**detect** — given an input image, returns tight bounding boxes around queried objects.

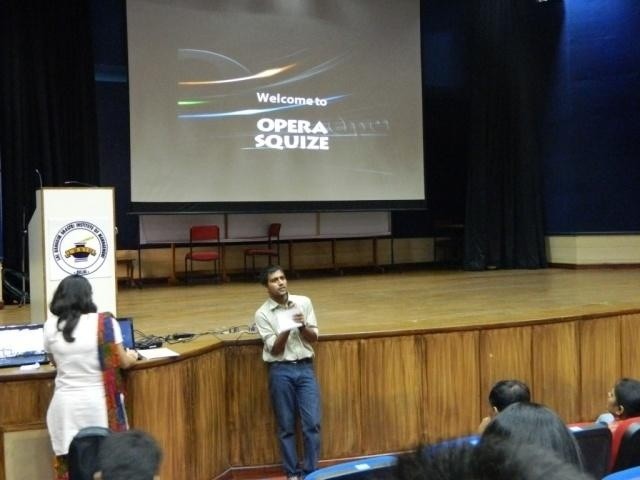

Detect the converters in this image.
[172,331,193,340]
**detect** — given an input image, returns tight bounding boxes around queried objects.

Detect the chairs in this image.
[306,456,397,480]
[184,225,221,280]
[612,414,639,472]
[243,222,281,277]
[565,421,610,478]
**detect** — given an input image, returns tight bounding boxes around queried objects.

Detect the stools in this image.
[117,258,135,286]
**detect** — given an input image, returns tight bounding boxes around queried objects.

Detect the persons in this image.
[480,402,585,471]
[366,433,598,480]
[93,429,163,480]
[254,264,321,480]
[44,275,138,480]
[607,378,640,437]
[478,380,531,432]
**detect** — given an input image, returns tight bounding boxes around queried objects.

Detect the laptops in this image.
[111,316,136,361]
[0,325,53,368]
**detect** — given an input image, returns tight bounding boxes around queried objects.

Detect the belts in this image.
[283,359,312,364]
